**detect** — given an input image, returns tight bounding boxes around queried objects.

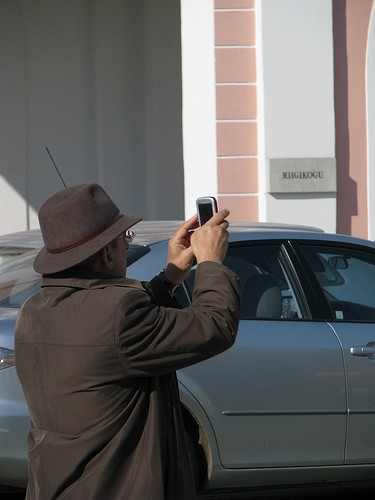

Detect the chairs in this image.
[185,269,196,303]
[242,274,283,318]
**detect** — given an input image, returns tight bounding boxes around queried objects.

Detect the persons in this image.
[13,184,242,499]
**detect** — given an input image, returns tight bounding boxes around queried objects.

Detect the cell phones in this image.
[196,197,217,227]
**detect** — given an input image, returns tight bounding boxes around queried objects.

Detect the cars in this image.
[0,219,375,500]
